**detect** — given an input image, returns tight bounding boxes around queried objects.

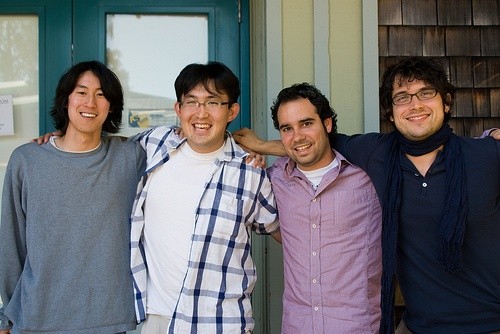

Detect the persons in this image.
[174,83,500,334]
[233,57,500,334]
[0,61,266,334]
[31,61,283,334]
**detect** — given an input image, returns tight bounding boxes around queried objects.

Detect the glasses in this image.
[392,88,438,106]
[179,99,230,112]
